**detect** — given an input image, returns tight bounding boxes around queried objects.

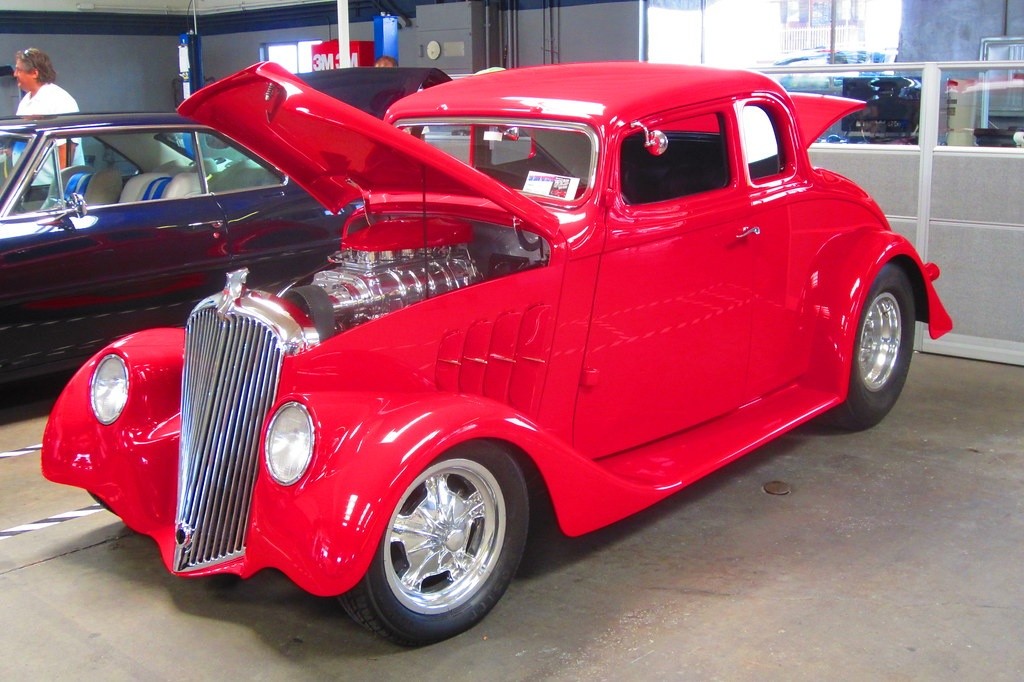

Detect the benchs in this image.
[621,129,726,204]
[158,157,267,192]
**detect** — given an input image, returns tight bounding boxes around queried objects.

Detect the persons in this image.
[0,46,86,202]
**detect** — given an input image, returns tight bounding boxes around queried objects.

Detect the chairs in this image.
[119,172,204,204]
[39,167,124,210]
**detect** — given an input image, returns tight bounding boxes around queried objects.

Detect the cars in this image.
[41,55,953,649]
[0,64,597,389]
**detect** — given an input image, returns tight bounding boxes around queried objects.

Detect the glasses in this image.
[24,49,36,68]
